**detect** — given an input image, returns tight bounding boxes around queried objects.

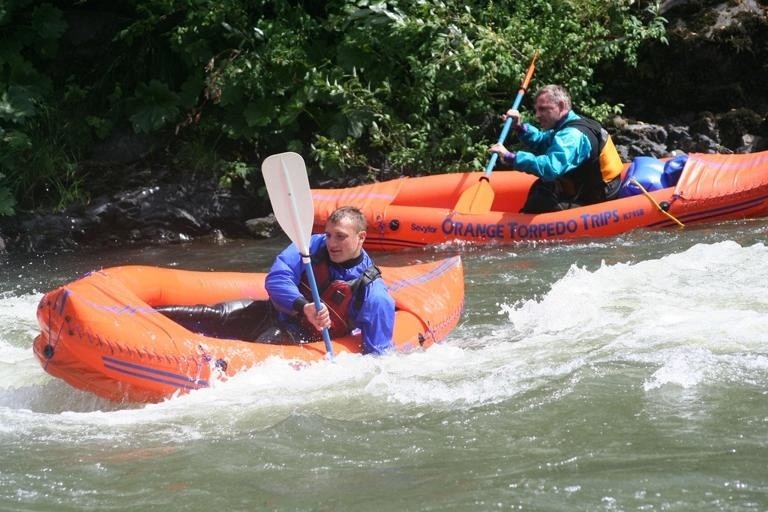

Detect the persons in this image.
[486,83,624,215]
[153,204,398,357]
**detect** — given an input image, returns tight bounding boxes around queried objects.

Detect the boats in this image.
[33,255,464,404]
[309,150,768,251]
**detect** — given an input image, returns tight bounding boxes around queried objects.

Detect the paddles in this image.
[261,152,334,361]
[454,53,538,212]
